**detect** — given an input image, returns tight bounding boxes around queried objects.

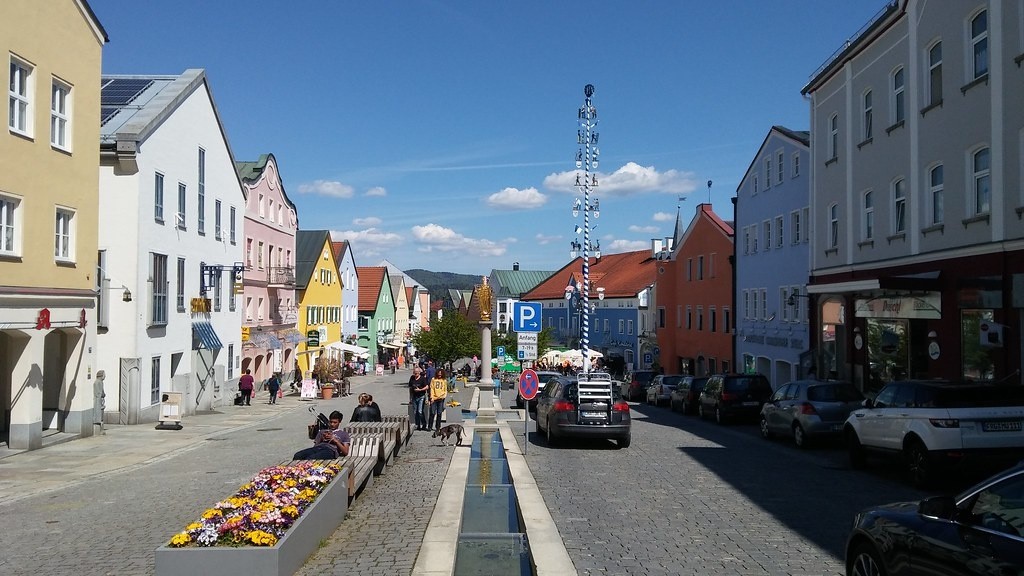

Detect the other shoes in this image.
[273,401,275,404]
[416,423,420,430]
[246,403,251,406]
[436,427,440,433]
[422,423,427,430]
[428,427,432,431]
[269,400,271,404]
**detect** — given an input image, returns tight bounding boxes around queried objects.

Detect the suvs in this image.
[697,372,775,425]
[625,369,664,403]
[843,376,1024,489]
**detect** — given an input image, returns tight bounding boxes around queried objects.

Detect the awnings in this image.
[388,342,407,348]
[332,343,370,355]
[260,332,282,347]
[354,352,371,359]
[278,330,301,344]
[193,323,225,351]
[380,344,398,350]
[290,328,310,343]
[248,335,271,350]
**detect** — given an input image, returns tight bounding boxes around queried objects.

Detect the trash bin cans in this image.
[158,390,182,425]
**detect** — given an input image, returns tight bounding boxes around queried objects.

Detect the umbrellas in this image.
[545,347,605,365]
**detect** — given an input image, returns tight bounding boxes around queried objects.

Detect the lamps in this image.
[109,285,131,301]
[787,293,808,306]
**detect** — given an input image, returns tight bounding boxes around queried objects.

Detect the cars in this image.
[647,374,687,407]
[516,370,563,409]
[669,376,710,416]
[534,376,630,448]
[845,460,1024,576]
[760,378,865,450]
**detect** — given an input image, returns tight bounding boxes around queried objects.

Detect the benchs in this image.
[280,414,415,505]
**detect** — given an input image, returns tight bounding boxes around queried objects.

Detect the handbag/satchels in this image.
[308,424,319,439]
[250,389,255,398]
[277,388,282,398]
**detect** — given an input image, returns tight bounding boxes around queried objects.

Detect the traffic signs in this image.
[497,346,505,356]
[513,302,542,333]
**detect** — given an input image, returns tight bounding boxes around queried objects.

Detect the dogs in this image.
[432,424,467,446]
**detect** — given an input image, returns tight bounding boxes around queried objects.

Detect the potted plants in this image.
[321,383,334,399]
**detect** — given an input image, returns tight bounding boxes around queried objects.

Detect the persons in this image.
[239,370,255,406]
[348,393,382,424]
[414,355,436,405]
[312,370,321,389]
[461,354,502,379]
[266,374,280,403]
[408,367,448,429]
[291,365,302,391]
[382,351,419,372]
[536,361,583,376]
[293,411,351,460]
